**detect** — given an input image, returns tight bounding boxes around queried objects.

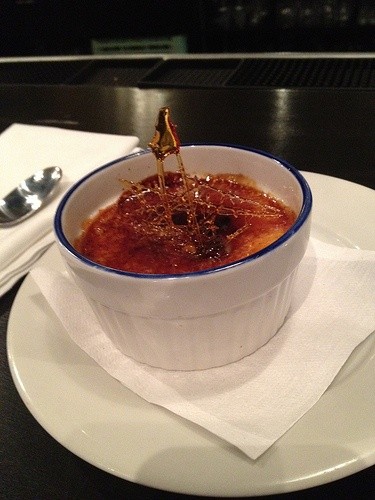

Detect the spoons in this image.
[0,166,61,228]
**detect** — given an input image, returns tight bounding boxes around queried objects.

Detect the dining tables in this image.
[0,76,375,500]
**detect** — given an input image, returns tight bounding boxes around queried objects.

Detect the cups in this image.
[56,139,314,375]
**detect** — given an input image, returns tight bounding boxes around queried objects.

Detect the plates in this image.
[6,166,374,500]
